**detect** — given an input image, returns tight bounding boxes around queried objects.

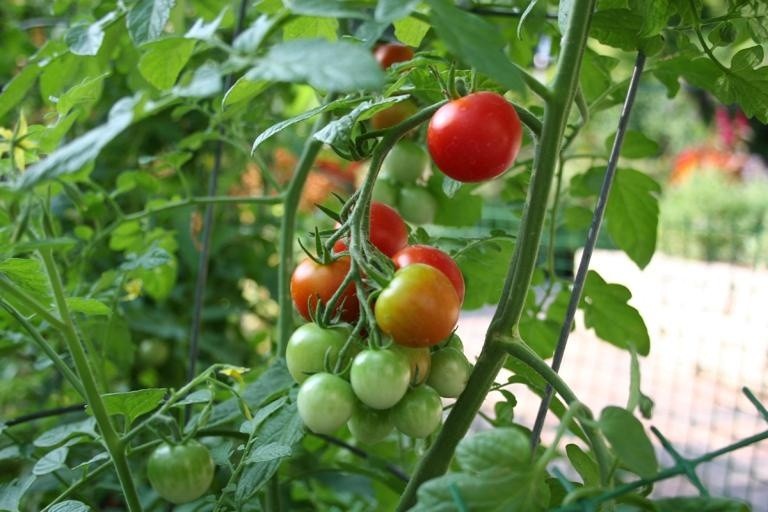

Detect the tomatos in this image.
[138,340,167,366]
[148,438,213,505]
[284,43,522,445]
